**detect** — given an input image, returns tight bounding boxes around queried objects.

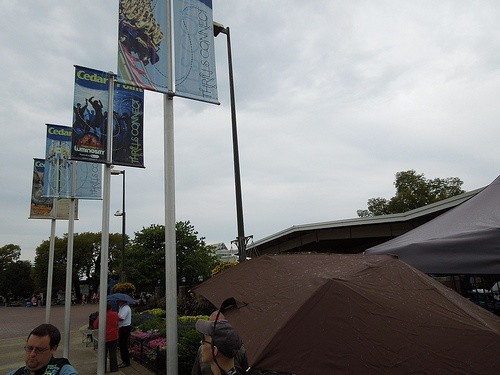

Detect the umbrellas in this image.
[188,254,500,375]
[107,294,135,305]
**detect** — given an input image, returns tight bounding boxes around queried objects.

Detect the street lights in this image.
[111,169,126,284]
[212,21,249,263]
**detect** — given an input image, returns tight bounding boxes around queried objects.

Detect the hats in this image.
[195,319,242,356]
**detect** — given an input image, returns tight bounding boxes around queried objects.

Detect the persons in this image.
[7,323,78,375]
[191,310,248,375]
[93,305,119,373]
[116,300,132,367]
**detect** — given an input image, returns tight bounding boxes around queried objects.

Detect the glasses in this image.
[24,343,51,353]
[202,335,216,346]
